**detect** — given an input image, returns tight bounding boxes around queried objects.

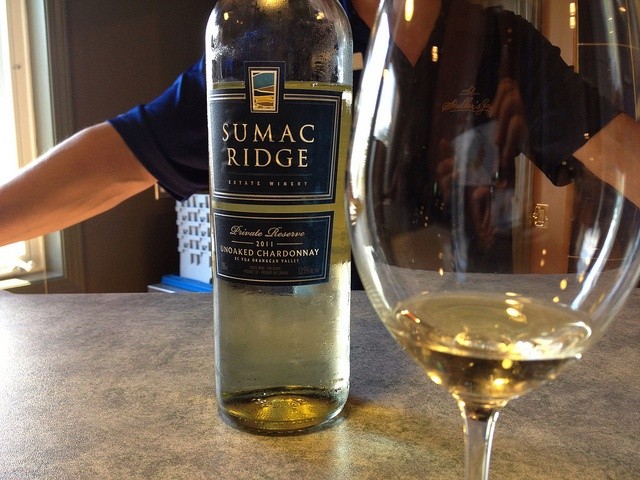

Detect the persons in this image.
[0,0,640,251]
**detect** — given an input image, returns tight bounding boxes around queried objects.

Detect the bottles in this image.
[205,0,352,440]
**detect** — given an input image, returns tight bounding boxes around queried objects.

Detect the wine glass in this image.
[344,0,640,480]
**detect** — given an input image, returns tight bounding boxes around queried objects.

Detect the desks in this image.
[1,290,640,480]
